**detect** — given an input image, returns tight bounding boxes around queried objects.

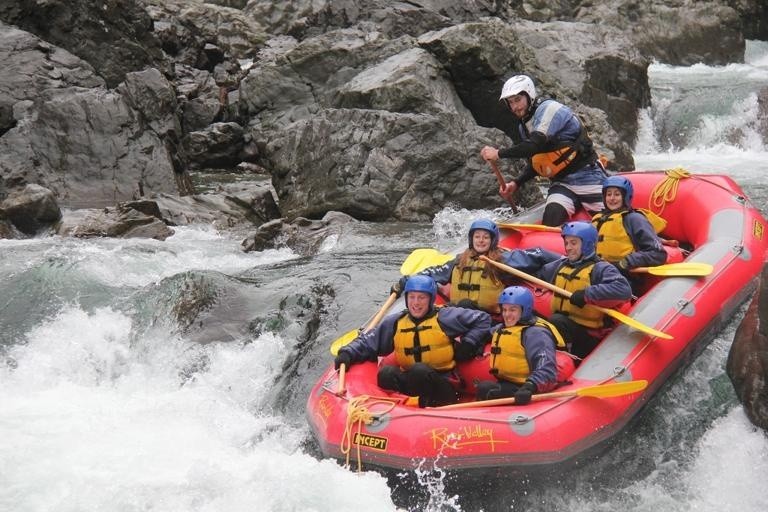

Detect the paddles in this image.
[363,248,440,333]
[329,256,452,357]
[627,263,714,277]
[498,223,678,248]
[480,256,673,341]
[425,380,647,409]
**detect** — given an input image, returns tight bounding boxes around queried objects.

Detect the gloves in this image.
[335,352,353,371]
[570,289,586,308]
[455,343,473,362]
[390,283,402,299]
[617,259,631,278]
[515,383,534,404]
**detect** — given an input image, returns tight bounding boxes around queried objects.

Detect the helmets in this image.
[561,221,598,257]
[468,219,499,251]
[499,75,537,104]
[602,176,634,208]
[405,275,437,312]
[498,285,534,320]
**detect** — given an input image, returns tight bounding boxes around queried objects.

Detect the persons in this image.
[586,172,667,281]
[477,72,614,229]
[334,272,493,406]
[475,283,560,408]
[384,216,525,313]
[494,220,631,346]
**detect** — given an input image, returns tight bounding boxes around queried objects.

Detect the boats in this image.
[305,171,768,491]
